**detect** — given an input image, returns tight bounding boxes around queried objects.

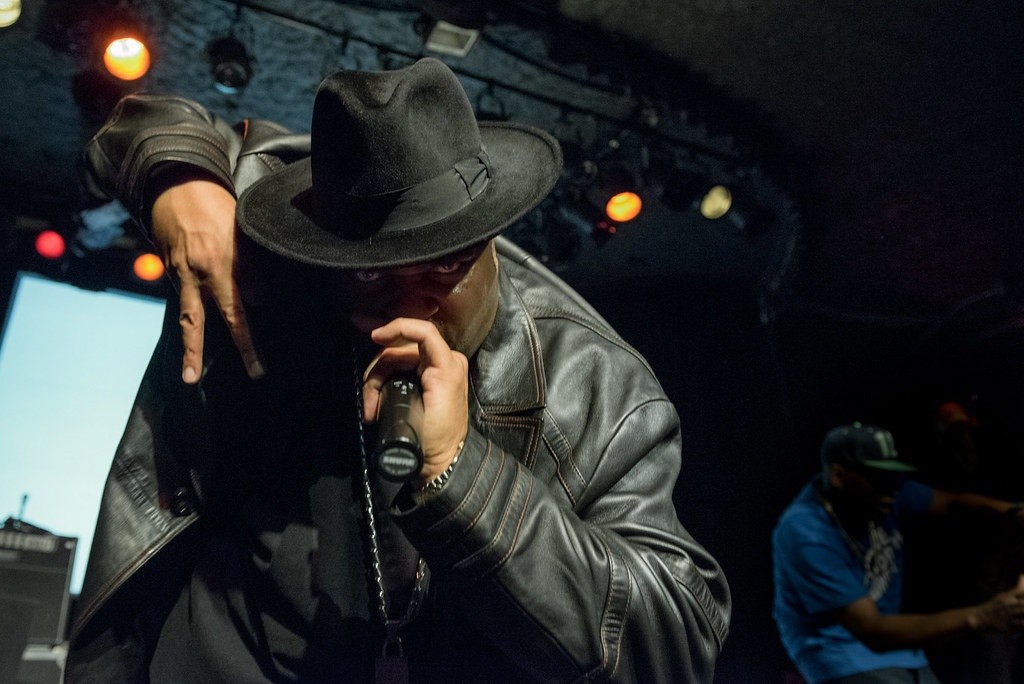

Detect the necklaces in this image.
[350,338,429,683]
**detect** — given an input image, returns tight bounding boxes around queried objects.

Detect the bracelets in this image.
[414,439,467,506]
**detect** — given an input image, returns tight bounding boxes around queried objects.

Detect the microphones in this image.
[372,370,427,484]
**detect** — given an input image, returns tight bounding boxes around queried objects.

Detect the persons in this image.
[768,423,1024,684]
[78,55,730,684]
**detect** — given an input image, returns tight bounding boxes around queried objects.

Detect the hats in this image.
[821,422,918,472]
[234,56,563,267]
